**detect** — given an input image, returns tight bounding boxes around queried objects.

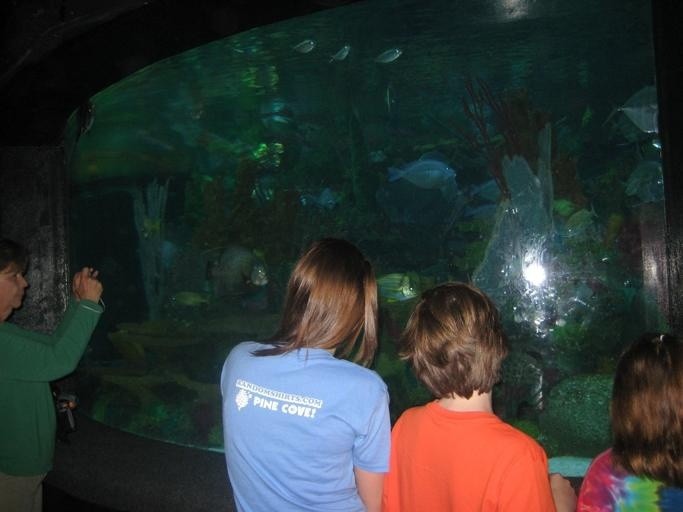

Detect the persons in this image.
[219,237,392,512]
[381,283,578,512]
[575,332,683,512]
[0,239,105,512]
